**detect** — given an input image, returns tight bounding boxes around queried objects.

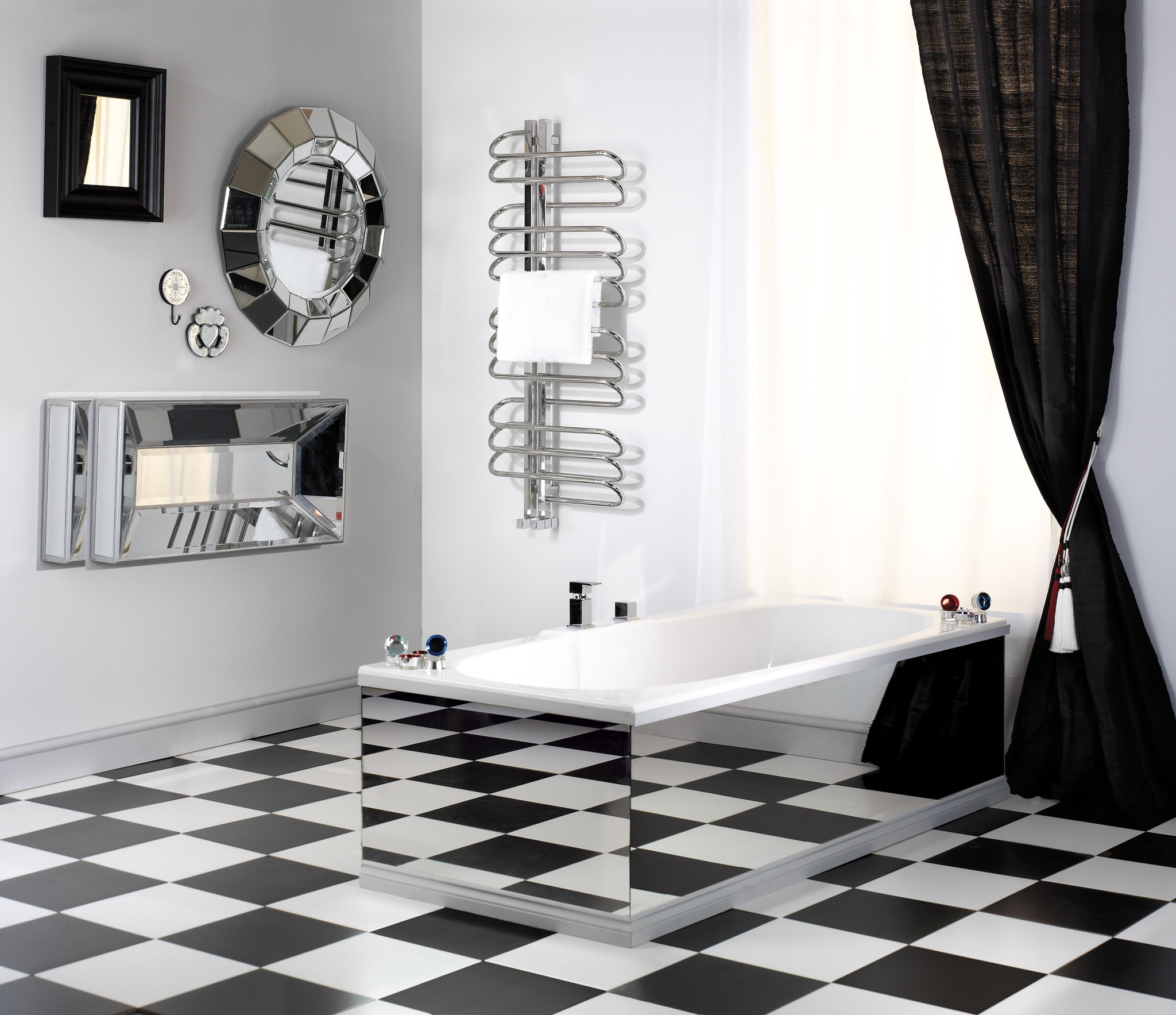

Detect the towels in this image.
[496,269,600,364]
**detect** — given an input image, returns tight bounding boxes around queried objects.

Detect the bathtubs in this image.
[359,595,1005,947]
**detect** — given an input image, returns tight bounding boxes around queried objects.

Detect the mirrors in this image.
[44,55,166,224]
[219,107,389,347]
